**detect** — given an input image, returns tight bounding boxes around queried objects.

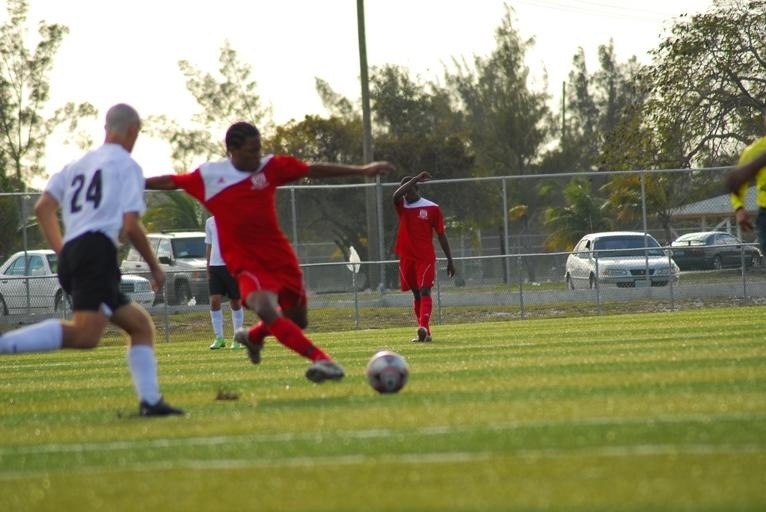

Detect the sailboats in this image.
[366,351,411,391]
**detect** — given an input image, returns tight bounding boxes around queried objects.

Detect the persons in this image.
[726,135,766,258]
[200,215,243,350]
[389,171,456,343]
[131,119,396,386]
[0,101,183,417]
[725,153,766,196]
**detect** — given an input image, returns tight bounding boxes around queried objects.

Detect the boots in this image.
[116,228,228,308]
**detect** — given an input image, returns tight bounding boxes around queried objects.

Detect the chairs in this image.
[210,338,224,349]
[411,328,433,342]
[231,326,263,363]
[139,397,183,416]
[307,359,343,383]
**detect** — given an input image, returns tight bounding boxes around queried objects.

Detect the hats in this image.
[661,229,762,273]
[0,245,159,318]
[562,230,681,290]
